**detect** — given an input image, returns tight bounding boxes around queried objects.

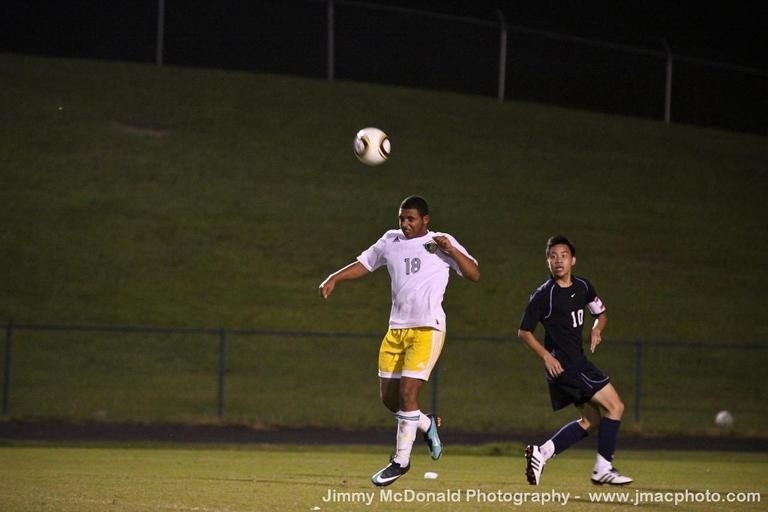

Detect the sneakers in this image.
[371,458,411,488]
[591,467,633,485]
[423,413,444,460]
[525,444,546,486]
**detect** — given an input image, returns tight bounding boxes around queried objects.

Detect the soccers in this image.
[352,127,391,168]
[715,410,734,428]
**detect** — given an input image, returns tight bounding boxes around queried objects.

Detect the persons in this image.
[318,195,482,488]
[515,232,637,486]
